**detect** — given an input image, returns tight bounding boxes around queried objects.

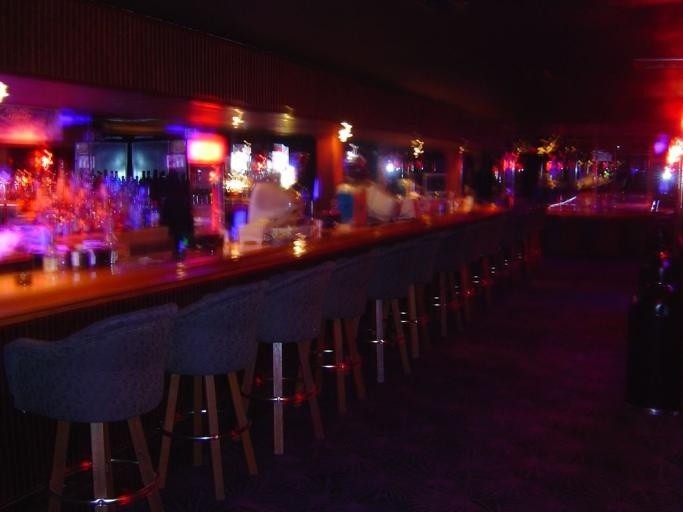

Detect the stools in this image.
[153,281,268,501]
[351,216,525,383]
[2,301,181,510]
[243,264,335,456]
[293,253,379,411]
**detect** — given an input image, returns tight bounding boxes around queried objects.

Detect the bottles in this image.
[90,165,169,206]
